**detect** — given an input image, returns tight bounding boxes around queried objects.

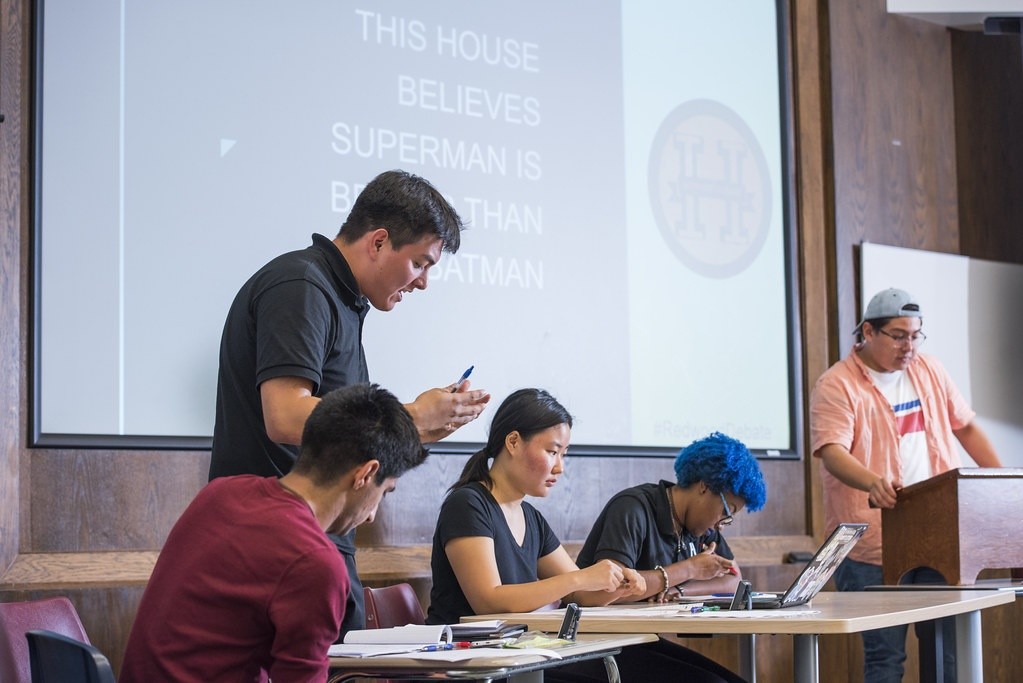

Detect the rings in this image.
[446,422,452,431]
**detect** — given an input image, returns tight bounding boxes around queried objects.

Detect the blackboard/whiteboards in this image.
[859,240,1023,468]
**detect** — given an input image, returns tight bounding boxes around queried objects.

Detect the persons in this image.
[208,171,490,630]
[120,383,425,683]
[808,289,1001,683]
[574,432,765,683]
[425,389,648,683]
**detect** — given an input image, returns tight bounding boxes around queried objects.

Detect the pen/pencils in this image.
[421,644,454,651]
[701,544,739,576]
[450,366,474,391]
[691,606,721,614]
[453,642,471,648]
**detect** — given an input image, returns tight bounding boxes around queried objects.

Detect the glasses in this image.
[714,484,734,527]
[872,324,927,349]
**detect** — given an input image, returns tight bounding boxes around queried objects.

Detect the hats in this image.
[852,287,923,336]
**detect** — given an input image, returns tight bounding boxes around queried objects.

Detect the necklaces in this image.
[666,488,681,554]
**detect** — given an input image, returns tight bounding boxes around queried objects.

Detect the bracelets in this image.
[655,565,669,595]
[675,585,685,596]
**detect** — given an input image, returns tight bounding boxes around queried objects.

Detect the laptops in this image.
[702,524,869,606]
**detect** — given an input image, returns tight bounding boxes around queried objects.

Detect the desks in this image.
[459,589,1016,683]
[322,632,658,683]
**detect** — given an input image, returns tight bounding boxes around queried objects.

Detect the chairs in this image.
[0,595,116,683]
[362,582,428,632]
